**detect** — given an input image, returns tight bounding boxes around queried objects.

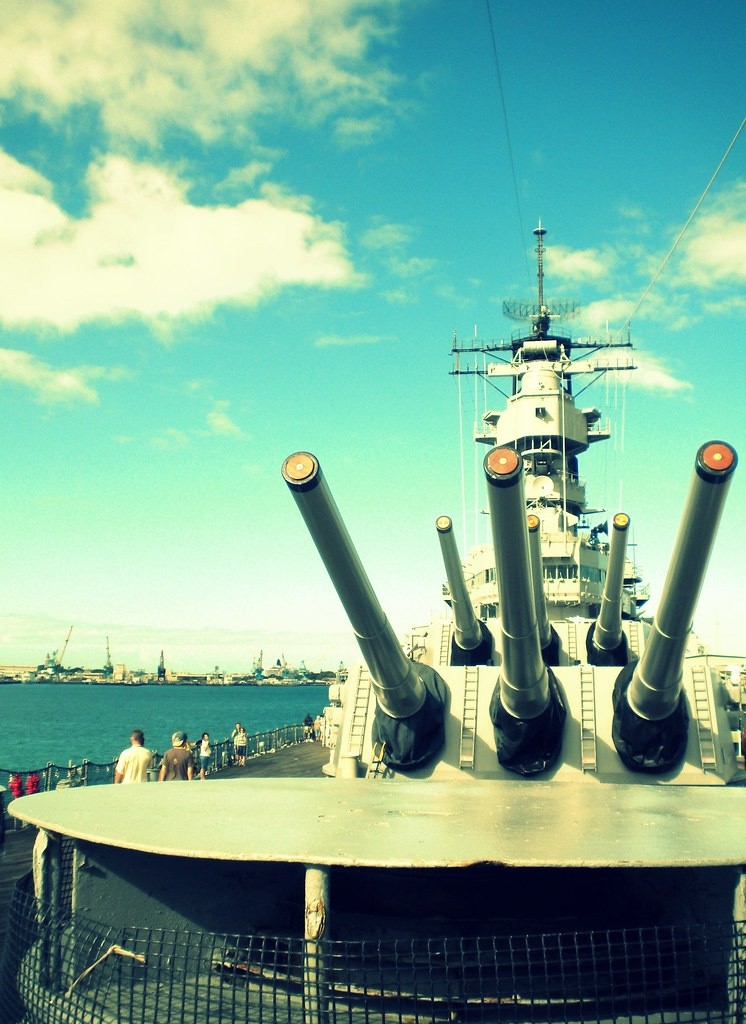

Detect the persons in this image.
[231,722,248,767]
[113,729,153,783]
[302,713,325,743]
[158,731,195,782]
[193,733,212,780]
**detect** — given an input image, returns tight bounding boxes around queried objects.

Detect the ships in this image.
[10,219,746,1024]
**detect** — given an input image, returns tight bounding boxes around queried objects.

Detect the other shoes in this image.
[233,762,246,768]
[305,738,313,743]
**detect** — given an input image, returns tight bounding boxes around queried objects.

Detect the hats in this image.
[171,731,187,748]
